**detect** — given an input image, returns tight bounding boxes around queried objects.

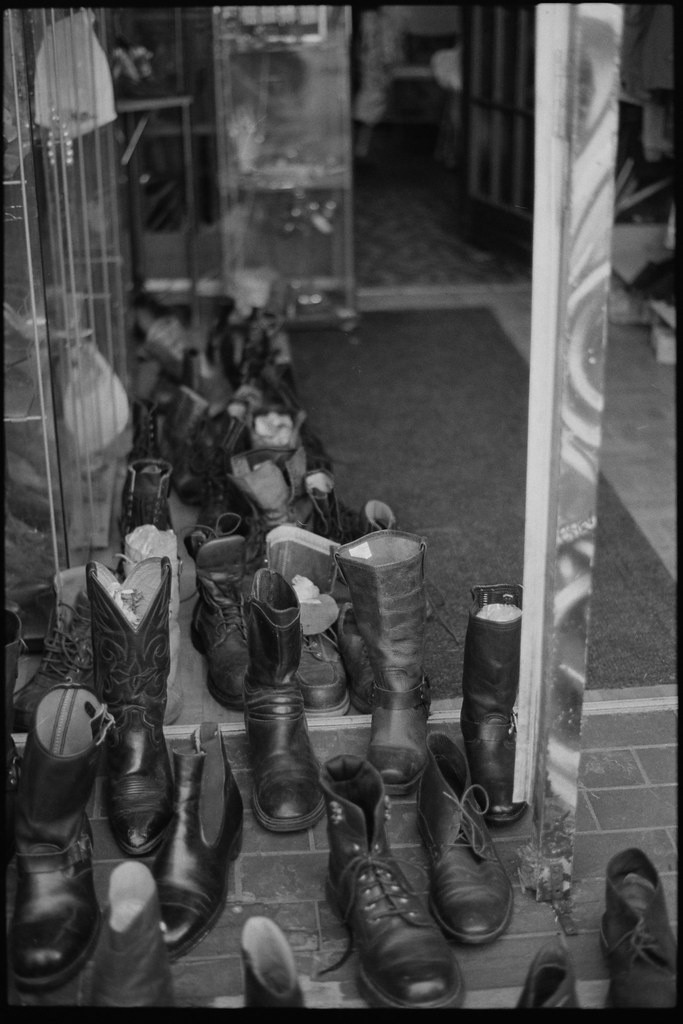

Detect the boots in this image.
[1,293,678,1009]
